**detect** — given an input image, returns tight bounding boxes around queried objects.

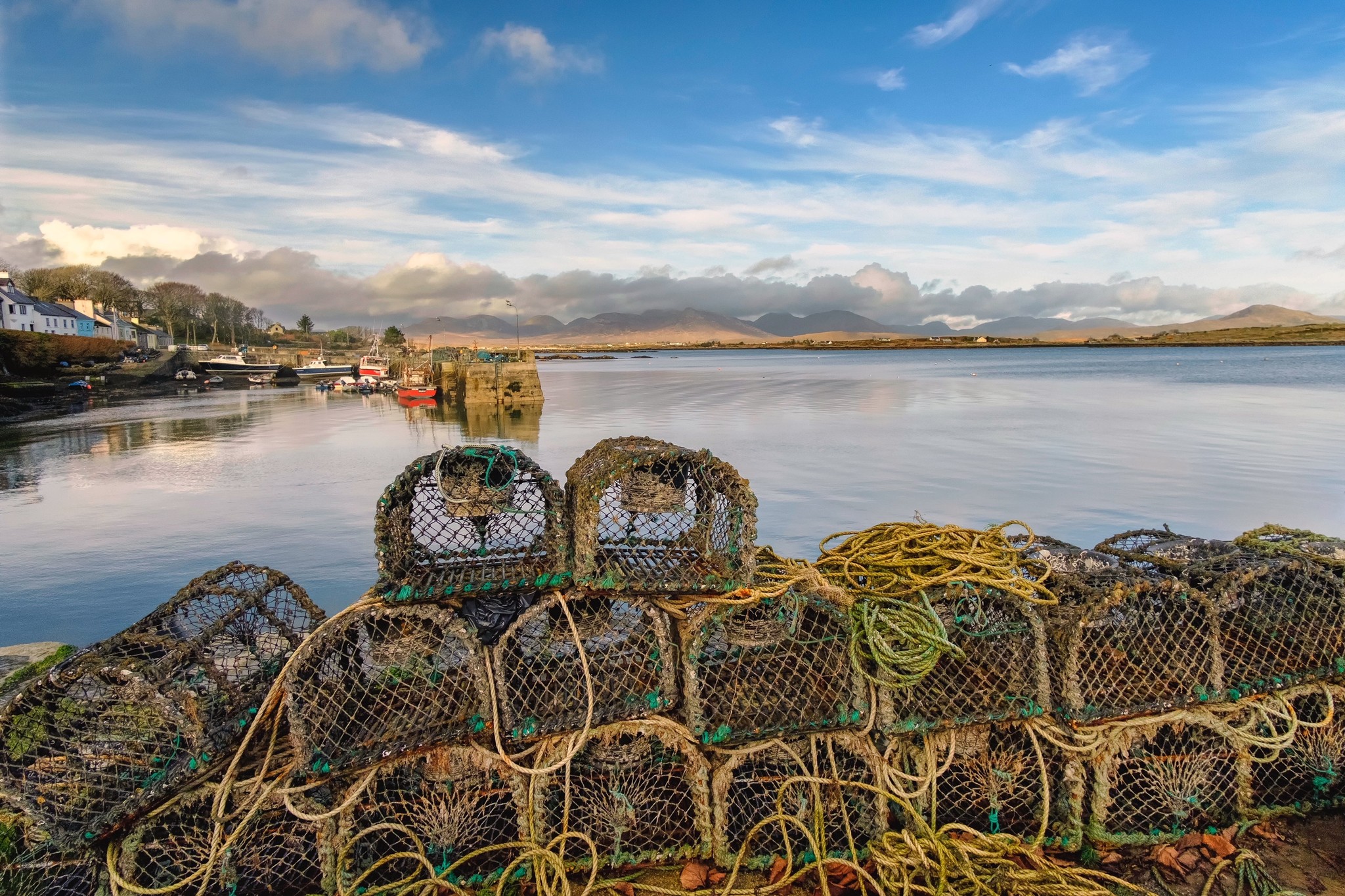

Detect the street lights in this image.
[436,317,447,351]
[506,299,520,350]
[401,324,410,350]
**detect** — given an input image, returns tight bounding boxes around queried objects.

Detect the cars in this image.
[177,344,189,350]
[137,355,147,363]
[198,344,211,351]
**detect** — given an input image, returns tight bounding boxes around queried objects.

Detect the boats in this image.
[332,376,358,392]
[208,376,223,385]
[395,334,437,398]
[290,339,354,380]
[357,319,390,379]
[356,376,379,394]
[174,367,197,380]
[315,378,334,390]
[68,380,92,390]
[378,380,398,392]
[196,354,286,378]
[247,372,275,383]
